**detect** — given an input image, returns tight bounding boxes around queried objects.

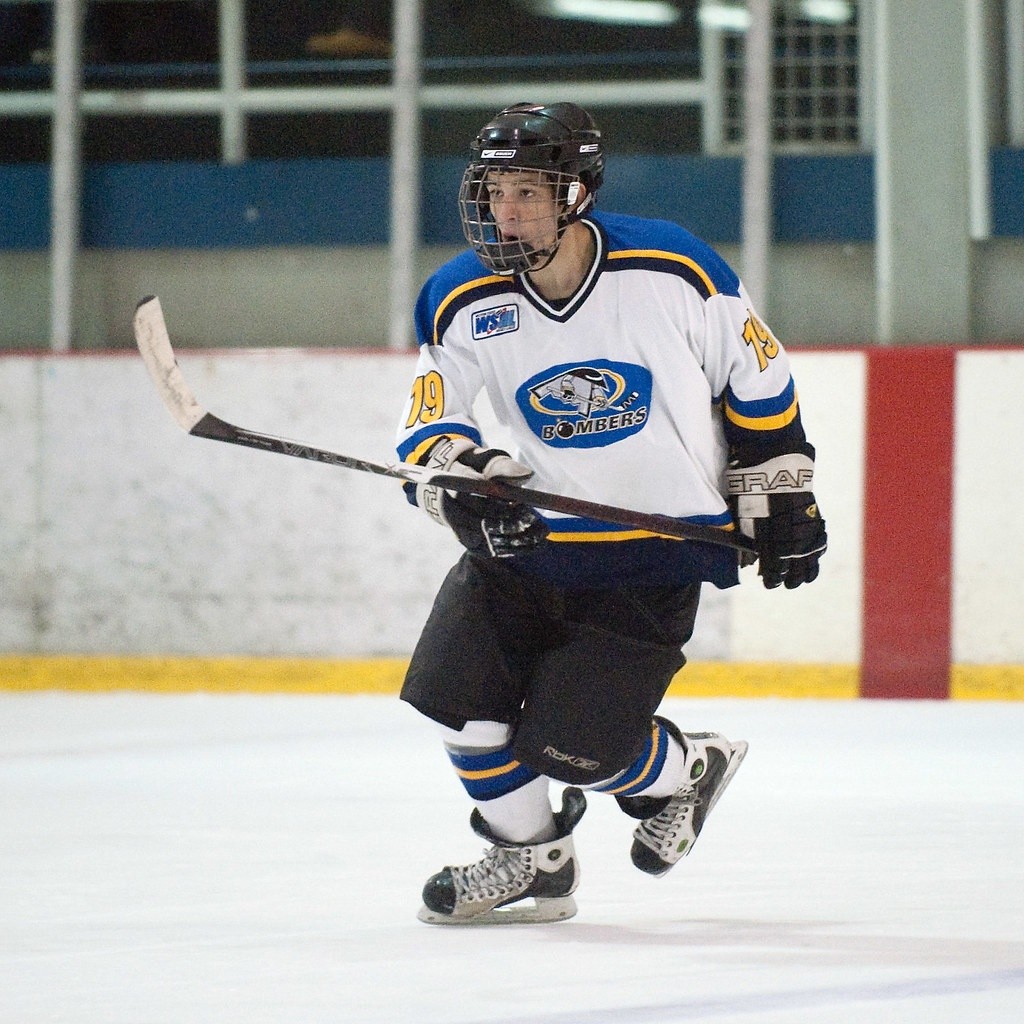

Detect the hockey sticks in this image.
[133,291,757,555]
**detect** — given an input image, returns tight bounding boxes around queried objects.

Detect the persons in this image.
[397,104,828,925]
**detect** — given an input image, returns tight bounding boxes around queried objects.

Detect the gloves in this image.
[726,488,827,589]
[415,443,551,562]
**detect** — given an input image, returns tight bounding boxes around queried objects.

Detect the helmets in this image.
[458,101,605,276]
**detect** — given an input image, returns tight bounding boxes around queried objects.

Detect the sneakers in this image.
[631,715,748,879]
[418,786,587,925]
